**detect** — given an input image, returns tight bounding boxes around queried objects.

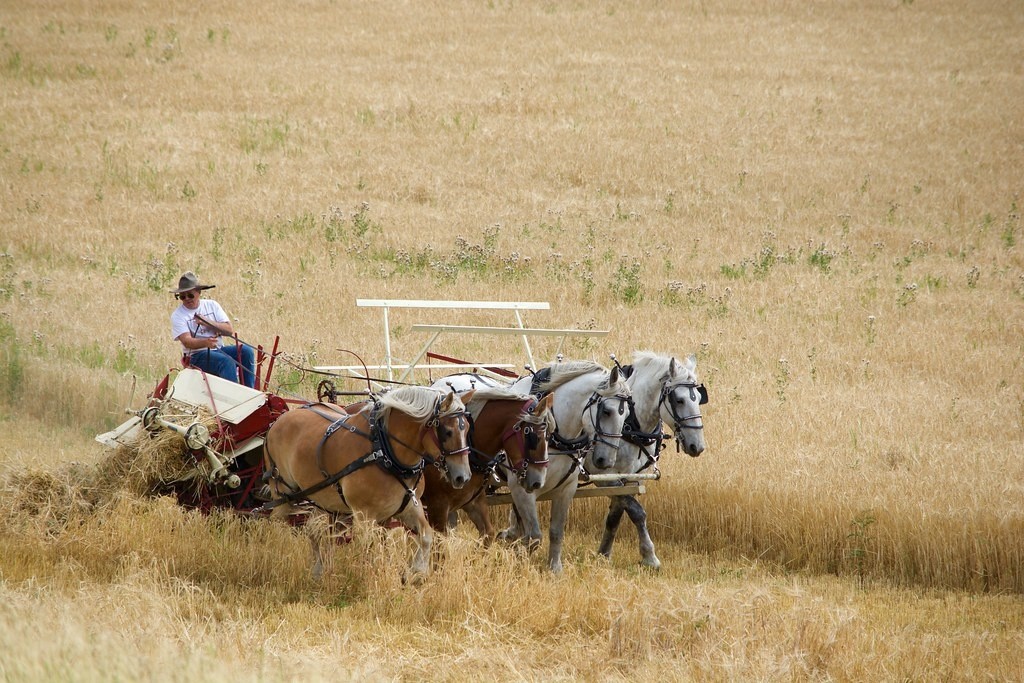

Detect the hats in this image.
[169,271,215,292]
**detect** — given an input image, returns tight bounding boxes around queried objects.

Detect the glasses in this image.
[179,292,198,300]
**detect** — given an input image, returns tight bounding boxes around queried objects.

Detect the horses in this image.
[263,351,705,588]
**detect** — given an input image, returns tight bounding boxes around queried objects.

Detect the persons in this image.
[170,271,255,389]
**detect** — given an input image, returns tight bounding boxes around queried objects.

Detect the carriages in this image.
[92,297,709,584]
